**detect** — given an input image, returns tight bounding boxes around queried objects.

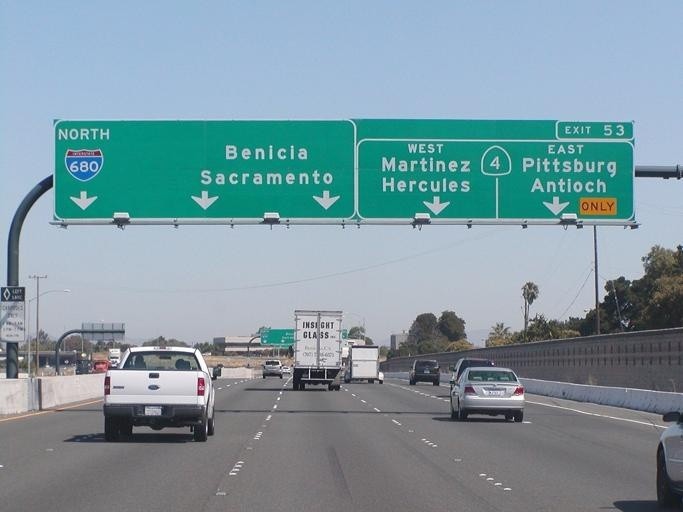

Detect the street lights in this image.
[26,285,70,301]
[27,273,49,375]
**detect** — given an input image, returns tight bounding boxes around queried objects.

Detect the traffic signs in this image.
[260,328,296,345]
[342,328,348,339]
[0,286,29,342]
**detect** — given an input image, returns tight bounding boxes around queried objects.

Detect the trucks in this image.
[341,345,381,384]
[1,343,122,377]
[294,309,344,391]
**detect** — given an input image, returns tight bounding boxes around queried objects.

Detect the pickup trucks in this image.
[99,346,222,442]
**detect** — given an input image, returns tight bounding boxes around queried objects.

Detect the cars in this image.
[282,364,291,375]
[654,407,683,512]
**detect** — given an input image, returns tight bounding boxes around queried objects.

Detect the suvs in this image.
[405,352,527,423]
[260,359,284,378]
